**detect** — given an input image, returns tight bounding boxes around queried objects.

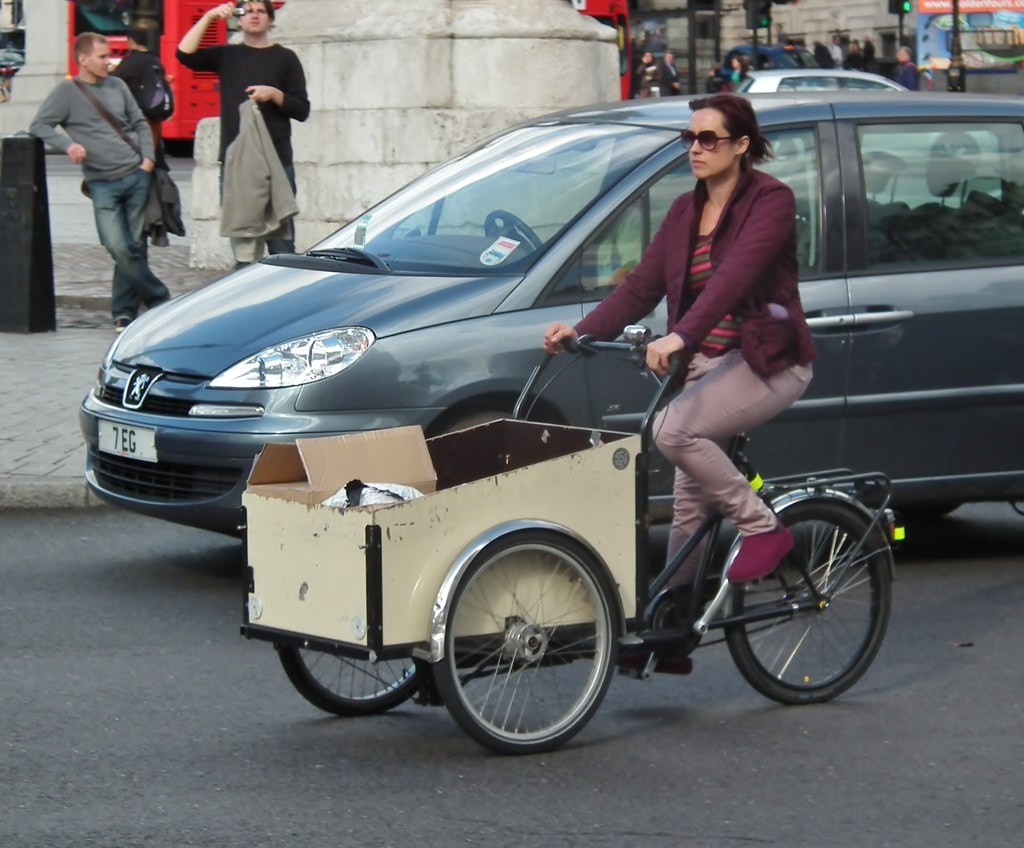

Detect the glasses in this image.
[680,128,733,151]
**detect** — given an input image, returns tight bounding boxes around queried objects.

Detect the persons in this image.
[179,0,310,271]
[627,26,921,96]
[32,29,175,332]
[537,95,819,676]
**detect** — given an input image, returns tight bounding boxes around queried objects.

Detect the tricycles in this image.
[235,322,907,758]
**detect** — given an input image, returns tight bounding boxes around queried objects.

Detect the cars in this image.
[719,39,822,83]
[70,86,1024,563]
[731,67,1001,181]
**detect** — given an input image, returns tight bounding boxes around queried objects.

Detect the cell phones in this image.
[233,8,245,17]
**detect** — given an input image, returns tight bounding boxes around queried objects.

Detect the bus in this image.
[61,0,637,158]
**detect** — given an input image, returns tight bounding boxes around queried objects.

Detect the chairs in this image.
[861,131,1024,264]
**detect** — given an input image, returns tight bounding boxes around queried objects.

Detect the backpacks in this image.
[130,51,174,121]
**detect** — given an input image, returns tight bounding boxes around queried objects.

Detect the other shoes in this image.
[113,317,133,332]
[727,526,795,583]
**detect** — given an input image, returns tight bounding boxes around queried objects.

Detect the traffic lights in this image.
[888,0,910,14]
[744,0,773,29]
